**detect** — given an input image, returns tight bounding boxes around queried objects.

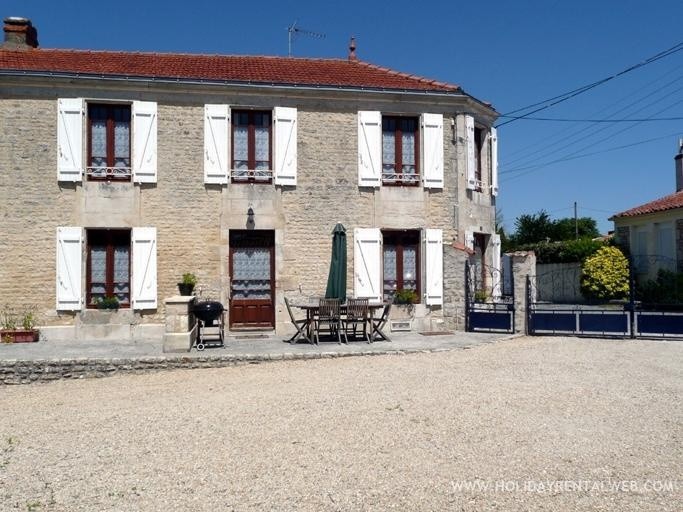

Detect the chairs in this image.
[283,295,395,344]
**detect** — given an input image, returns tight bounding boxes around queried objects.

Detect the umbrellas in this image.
[324,222,347,304]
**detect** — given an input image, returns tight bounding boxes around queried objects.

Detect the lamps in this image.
[245,207,255,224]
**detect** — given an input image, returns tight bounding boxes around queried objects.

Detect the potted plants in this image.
[0,307,40,345]
[175,273,197,296]
